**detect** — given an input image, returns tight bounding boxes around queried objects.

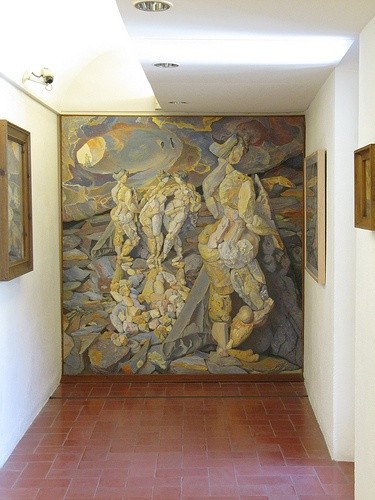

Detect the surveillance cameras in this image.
[41,67,53,84]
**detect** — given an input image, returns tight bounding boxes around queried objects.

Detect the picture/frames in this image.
[0,119,34,281]
[354,144,375,231]
[303,150,326,285]
